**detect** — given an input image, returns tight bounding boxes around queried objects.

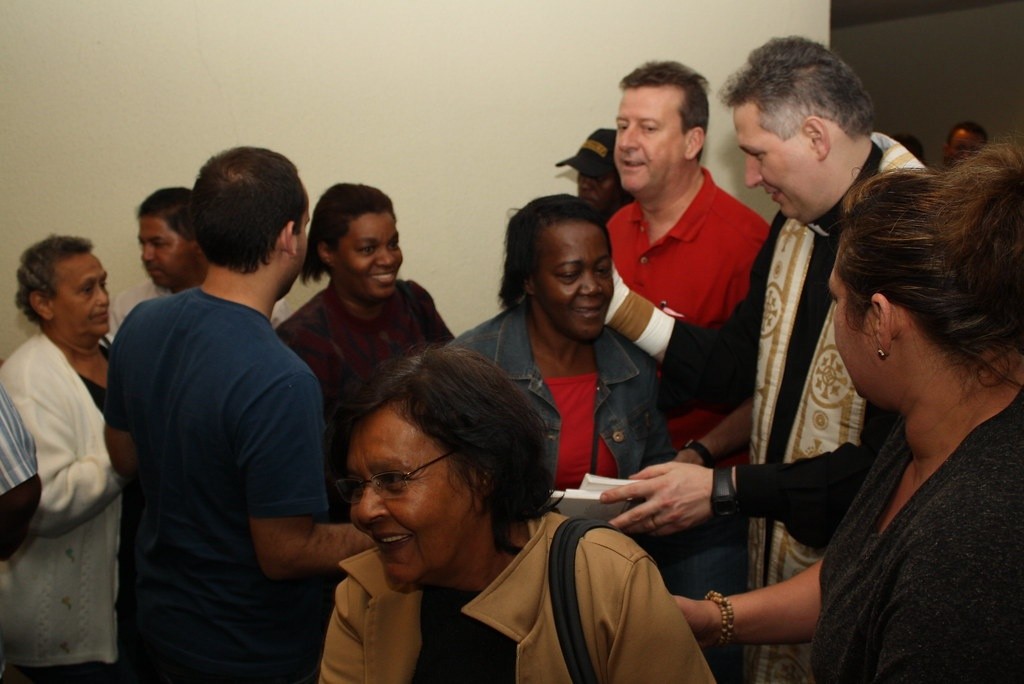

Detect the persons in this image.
[892,131,924,163]
[0,234,126,684]
[274,184,456,500]
[136,187,198,296]
[557,130,633,218]
[605,60,770,684]
[600,36,927,683]
[102,146,377,684]
[945,124,987,167]
[452,193,675,501]
[319,348,715,684]
[675,147,1024,684]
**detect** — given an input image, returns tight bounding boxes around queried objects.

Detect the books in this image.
[548,473,645,522]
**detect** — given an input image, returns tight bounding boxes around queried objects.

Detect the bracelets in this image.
[705,591,734,649]
[682,439,713,469]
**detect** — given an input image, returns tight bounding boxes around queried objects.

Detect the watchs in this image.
[710,466,739,520]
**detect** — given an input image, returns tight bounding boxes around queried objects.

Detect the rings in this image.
[651,515,660,529]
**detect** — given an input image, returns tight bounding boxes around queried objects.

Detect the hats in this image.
[556,129,616,177]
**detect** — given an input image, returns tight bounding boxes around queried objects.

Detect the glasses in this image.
[335,452,452,501]
